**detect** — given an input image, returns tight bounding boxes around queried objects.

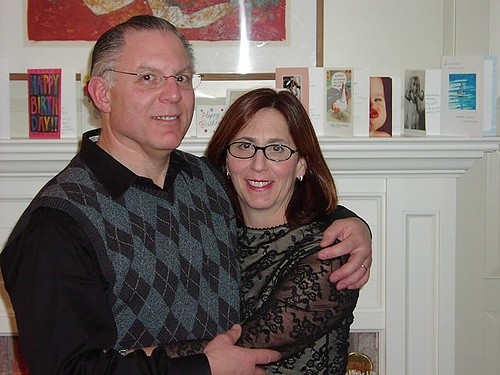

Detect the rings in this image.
[361,264,369,271]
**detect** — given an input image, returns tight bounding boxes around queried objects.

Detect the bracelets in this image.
[119,347,126,357]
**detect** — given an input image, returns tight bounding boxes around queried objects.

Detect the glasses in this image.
[101,68,204,90]
[226,141,298,162]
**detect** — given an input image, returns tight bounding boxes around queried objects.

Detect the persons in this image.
[116,88,360,375]
[369,78,391,137]
[0,15,372,375]
[406,77,425,131]
